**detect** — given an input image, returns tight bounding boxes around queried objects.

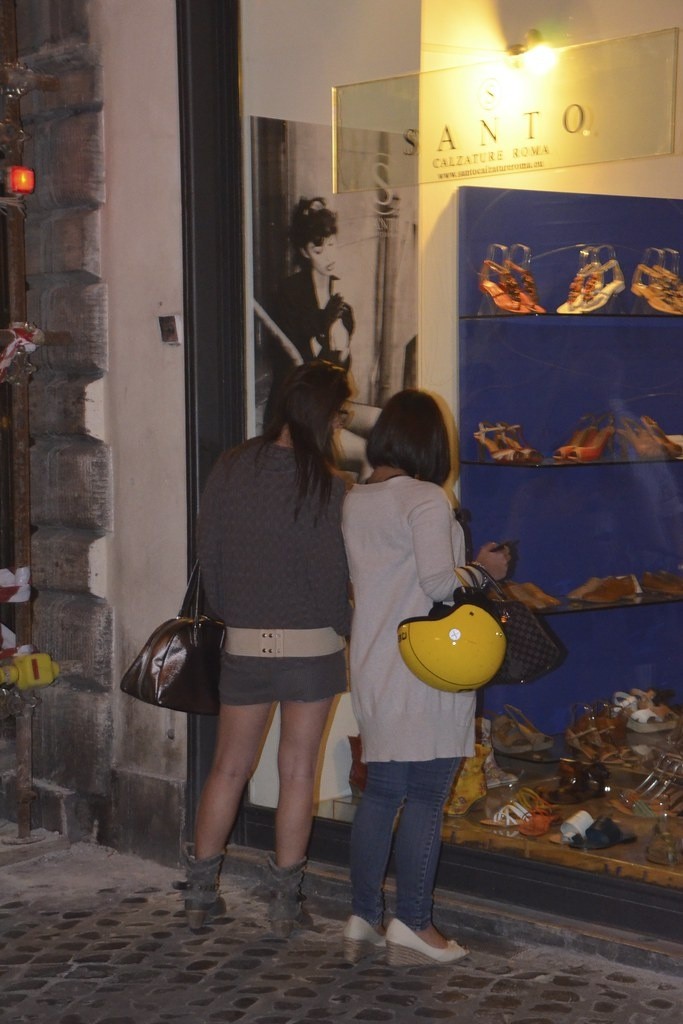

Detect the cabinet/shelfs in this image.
[454,185,683,782]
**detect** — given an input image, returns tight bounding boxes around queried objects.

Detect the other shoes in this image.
[476,688,683,866]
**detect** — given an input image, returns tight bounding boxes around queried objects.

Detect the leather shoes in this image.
[487,570,683,611]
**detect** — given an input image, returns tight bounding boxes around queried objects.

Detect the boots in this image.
[346,737,367,800]
[472,716,517,791]
[184,840,226,929]
[444,743,492,817]
[264,854,308,938]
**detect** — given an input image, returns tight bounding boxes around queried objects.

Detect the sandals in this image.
[579,259,625,313]
[479,260,531,314]
[499,260,546,315]
[556,262,604,315]
[631,264,683,316]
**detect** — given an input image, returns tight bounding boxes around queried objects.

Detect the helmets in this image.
[397,587,507,692]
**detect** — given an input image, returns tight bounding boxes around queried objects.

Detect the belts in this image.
[224,623,347,659]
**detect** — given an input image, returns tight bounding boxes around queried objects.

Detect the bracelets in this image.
[473,561,487,589]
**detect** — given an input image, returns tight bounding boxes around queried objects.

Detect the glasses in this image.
[334,408,349,426]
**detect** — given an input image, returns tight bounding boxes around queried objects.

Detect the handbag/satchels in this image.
[119,559,226,716]
[459,563,566,696]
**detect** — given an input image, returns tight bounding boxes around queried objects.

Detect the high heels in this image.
[343,915,384,964]
[471,414,682,466]
[385,917,471,968]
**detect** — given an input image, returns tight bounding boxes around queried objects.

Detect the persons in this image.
[341,388,511,968]
[262,197,385,482]
[185,358,352,943]
